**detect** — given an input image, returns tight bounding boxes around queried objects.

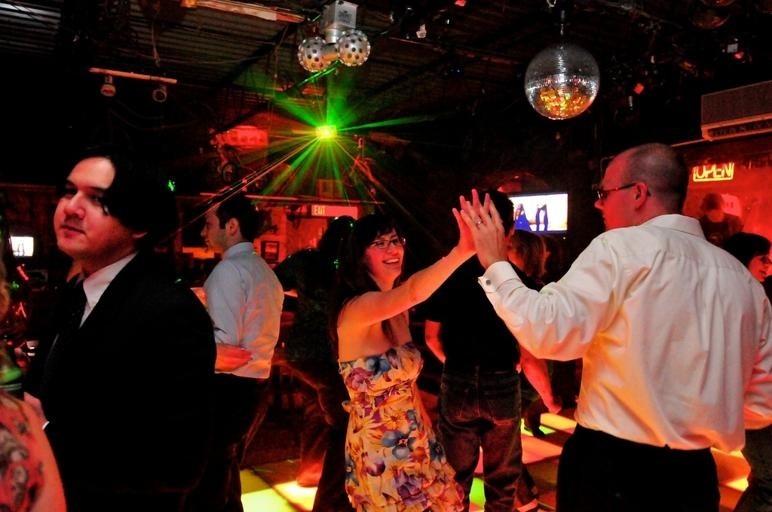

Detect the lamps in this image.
[90,64,182,109]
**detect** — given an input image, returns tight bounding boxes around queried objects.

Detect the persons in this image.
[200,195,285,500]
[272,186,561,512]
[0,144,245,512]
[461,142,772,512]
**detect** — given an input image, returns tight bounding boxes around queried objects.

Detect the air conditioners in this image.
[693,74,772,145]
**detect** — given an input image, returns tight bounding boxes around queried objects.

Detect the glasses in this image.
[370,236,406,248]
[596,182,651,199]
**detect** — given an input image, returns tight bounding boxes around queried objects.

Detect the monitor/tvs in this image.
[10,235,34,257]
[508,190,572,243]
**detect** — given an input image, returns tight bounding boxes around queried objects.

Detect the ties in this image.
[52,281,87,349]
